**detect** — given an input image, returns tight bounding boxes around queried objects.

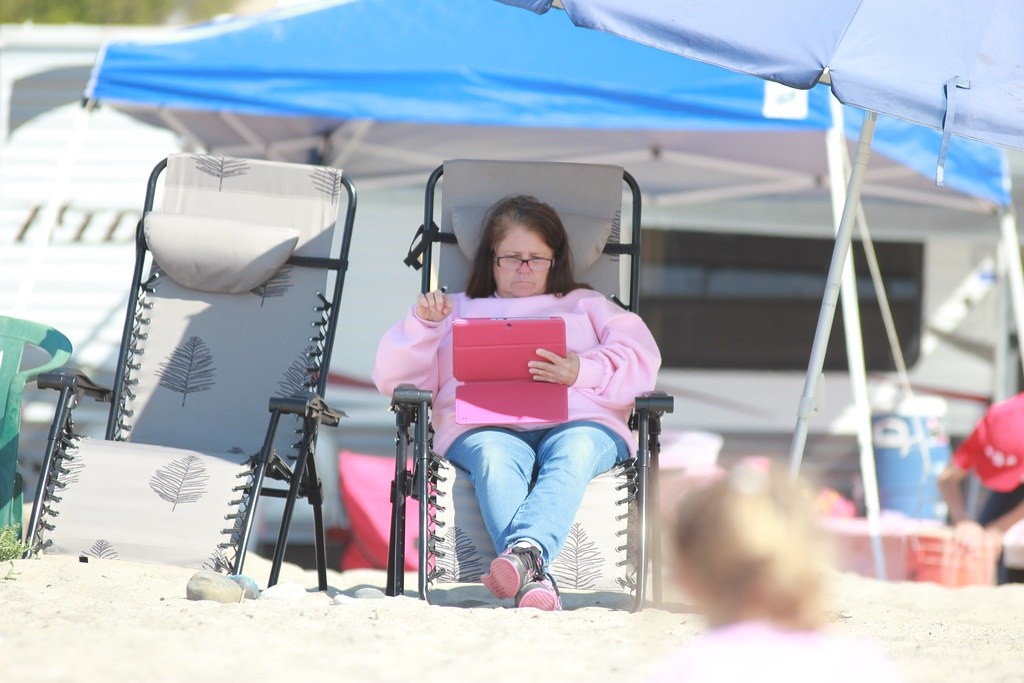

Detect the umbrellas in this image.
[487,0,1023,489]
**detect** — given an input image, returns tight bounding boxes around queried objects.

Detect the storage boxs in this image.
[906,535,997,589]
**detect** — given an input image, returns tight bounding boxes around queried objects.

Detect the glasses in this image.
[492,252,555,271]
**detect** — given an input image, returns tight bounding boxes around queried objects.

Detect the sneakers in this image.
[515,572,563,611]
[479,545,545,599]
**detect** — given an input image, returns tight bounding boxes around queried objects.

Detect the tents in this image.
[79,0,1024,585]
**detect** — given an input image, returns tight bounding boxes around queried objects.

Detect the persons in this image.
[371,193,663,611]
[941,389,1024,586]
[663,458,908,683]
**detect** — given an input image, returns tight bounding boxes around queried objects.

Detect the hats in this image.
[976,392,1023,492]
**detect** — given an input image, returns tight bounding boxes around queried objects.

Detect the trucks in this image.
[0,21,1024,548]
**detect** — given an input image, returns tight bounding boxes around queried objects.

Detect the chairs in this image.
[0,315,72,540]
[21,154,358,591]
[385,157,675,607]
